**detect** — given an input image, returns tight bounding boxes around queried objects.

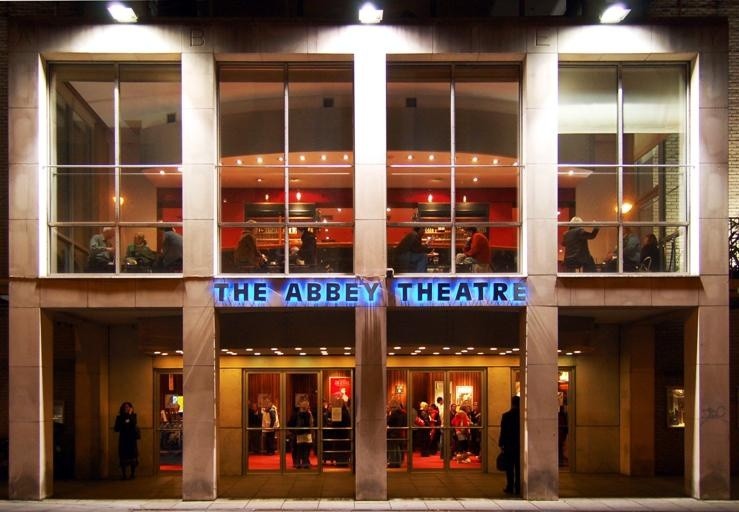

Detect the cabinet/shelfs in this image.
[421,200,488,243]
[245,202,318,240]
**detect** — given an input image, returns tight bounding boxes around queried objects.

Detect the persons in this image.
[124,232,149,271]
[387,228,433,273]
[249,395,482,469]
[86,227,115,272]
[114,402,141,481]
[561,216,600,272]
[456,227,491,273]
[295,227,321,272]
[159,228,182,273]
[497,396,520,496]
[640,230,663,267]
[605,222,640,272]
[234,220,266,272]
